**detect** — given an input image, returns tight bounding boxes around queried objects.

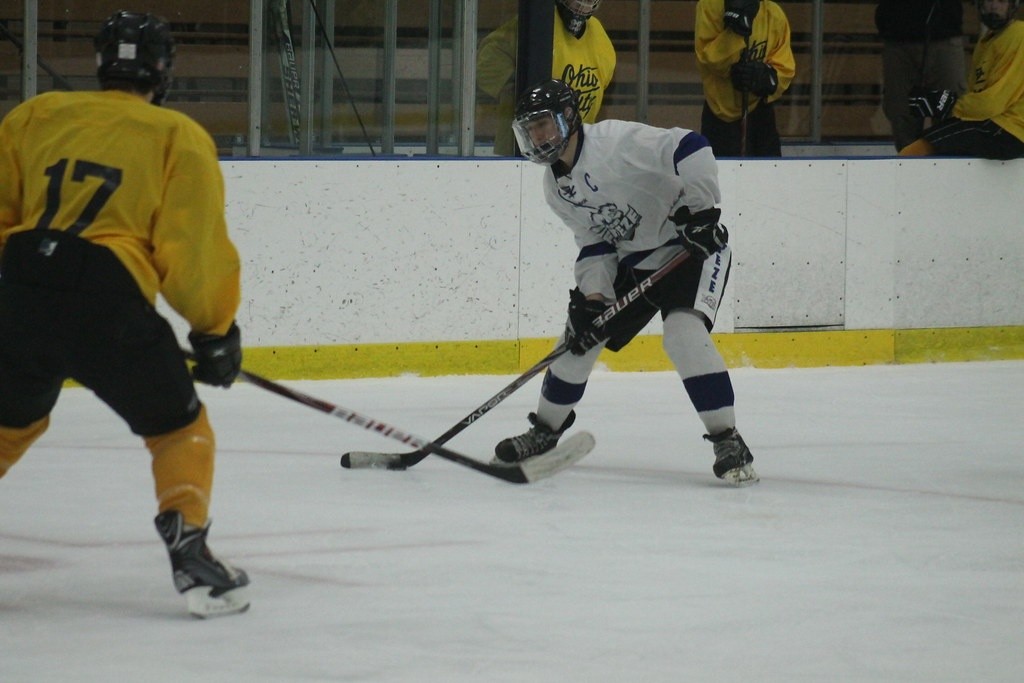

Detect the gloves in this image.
[723,0,760,35]
[732,61,778,96]
[188,321,242,388]
[564,289,610,356]
[677,208,729,262]
[907,87,958,124]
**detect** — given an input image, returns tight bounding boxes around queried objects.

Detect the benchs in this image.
[1,0,894,149]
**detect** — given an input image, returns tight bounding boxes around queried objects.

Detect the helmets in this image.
[512,80,581,165]
[557,0,601,19]
[93,13,174,93]
[977,0,1020,30]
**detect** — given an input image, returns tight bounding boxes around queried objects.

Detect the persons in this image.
[476,0,616,157]
[0,9,251,618]
[874,0,1024,160]
[694,0,795,157]
[493,74,760,483]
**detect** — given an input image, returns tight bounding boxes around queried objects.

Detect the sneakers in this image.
[491,409,576,467]
[703,426,758,487]
[154,509,251,618]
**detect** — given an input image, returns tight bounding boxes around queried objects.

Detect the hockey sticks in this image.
[735,36,751,156]
[180,345,599,487]
[339,247,693,473]
